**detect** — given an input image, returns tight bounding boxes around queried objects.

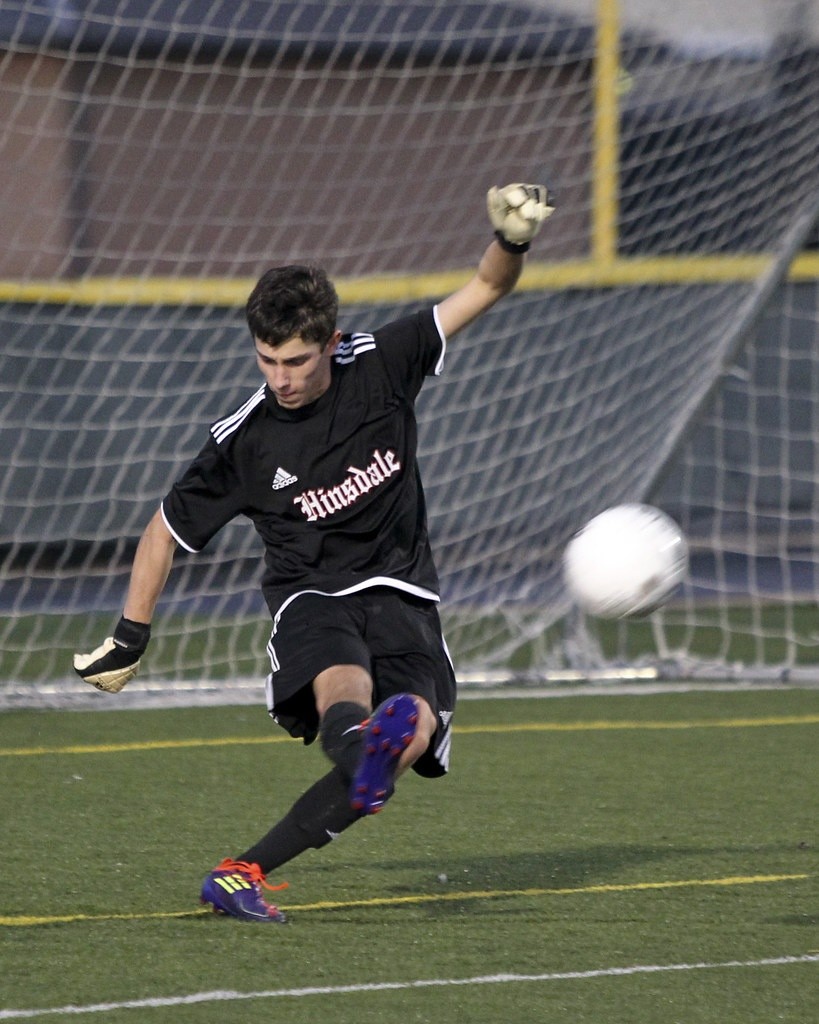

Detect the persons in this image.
[73,181,553,920]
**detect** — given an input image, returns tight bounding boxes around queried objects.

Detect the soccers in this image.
[561,502,691,622]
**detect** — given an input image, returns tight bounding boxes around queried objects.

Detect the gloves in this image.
[73,615,152,696]
[486,182,555,255]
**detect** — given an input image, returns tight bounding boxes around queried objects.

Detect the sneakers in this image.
[199,858,289,924]
[346,694,420,813]
